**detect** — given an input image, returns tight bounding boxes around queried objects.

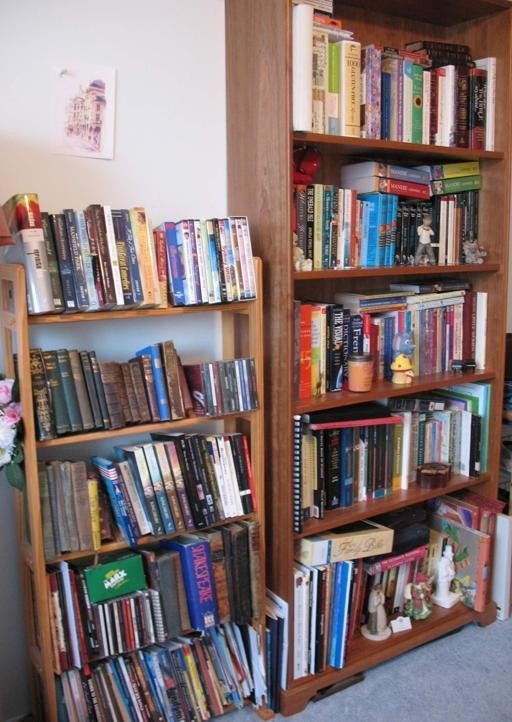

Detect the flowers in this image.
[0,374,25,493]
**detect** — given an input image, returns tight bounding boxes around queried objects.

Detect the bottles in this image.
[348,353,374,392]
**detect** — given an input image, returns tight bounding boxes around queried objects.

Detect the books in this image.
[294,380,491,532]
[292,0,497,152]
[294,279,488,401]
[1,192,257,314]
[50,518,283,722]
[294,490,498,680]
[39,432,255,561]
[296,161,481,269]
[14,339,258,442]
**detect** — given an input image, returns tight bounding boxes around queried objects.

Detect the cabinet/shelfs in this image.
[225,0,511,716]
[0,256,277,722]
